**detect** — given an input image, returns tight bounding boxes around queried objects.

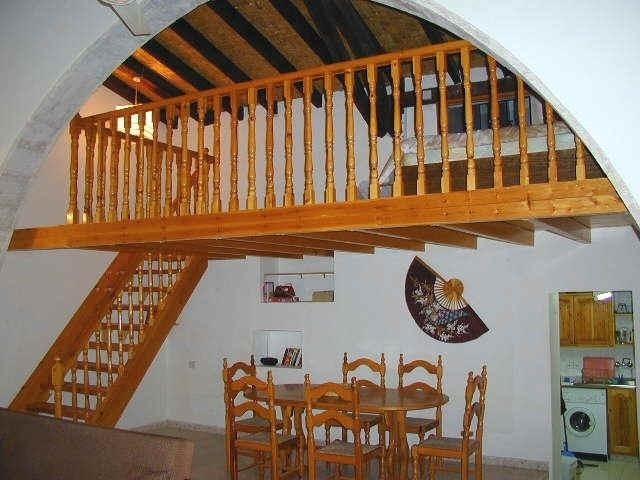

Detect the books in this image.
[281,347,302,368]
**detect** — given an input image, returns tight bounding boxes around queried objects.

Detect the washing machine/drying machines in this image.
[561,387,608,461]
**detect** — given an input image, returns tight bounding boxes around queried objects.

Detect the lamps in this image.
[115,76,154,140]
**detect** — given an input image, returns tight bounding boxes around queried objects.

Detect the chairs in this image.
[221,351,487,480]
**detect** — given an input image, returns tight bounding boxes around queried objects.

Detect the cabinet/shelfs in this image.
[560,293,616,349]
[607,388,639,457]
